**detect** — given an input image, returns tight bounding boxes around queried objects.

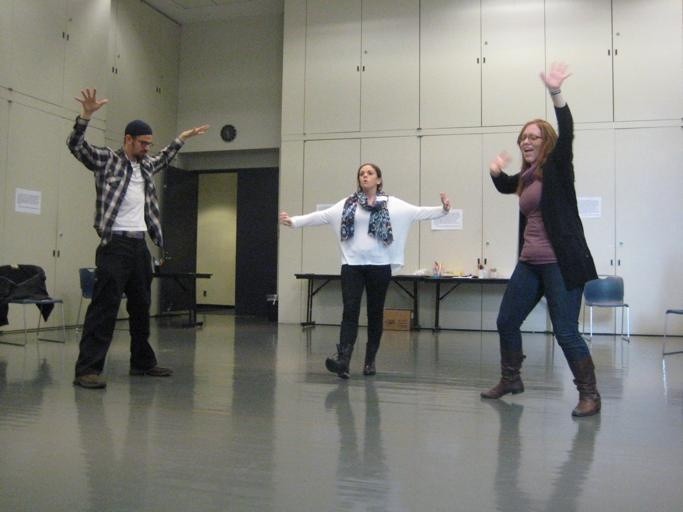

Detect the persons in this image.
[480,59,602,416]
[279,163,451,379]
[66,87,212,388]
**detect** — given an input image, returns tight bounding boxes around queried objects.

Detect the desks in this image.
[294,273,425,331]
[153,272,211,327]
[422,276,556,337]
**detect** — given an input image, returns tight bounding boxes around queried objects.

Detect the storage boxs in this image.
[382,308,414,331]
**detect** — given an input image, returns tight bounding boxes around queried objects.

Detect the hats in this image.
[123,120,152,137]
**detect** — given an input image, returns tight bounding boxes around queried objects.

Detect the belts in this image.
[103,229,145,240]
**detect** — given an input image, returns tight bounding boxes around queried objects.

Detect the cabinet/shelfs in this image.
[303,132,420,328]
[416,0,545,135]
[304,0,419,139]
[547,121,682,337]
[545,0,683,129]
[0,97,113,334]
[103,122,164,321]
[0,0,182,148]
[419,126,552,334]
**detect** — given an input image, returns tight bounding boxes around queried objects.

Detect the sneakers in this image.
[128,361,176,377]
[72,375,107,389]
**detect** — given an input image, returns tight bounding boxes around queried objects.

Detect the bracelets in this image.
[550,90,561,96]
[178,137,184,144]
[79,116,89,122]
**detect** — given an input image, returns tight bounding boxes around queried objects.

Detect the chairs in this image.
[0,264,66,346]
[581,274,631,343]
[662,309,683,355]
[76,267,127,338]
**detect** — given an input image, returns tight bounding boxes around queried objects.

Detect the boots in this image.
[566,355,602,418]
[324,343,353,379]
[361,342,380,375]
[478,349,526,399]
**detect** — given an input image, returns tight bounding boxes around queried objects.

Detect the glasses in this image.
[133,139,153,149]
[518,133,543,141]
